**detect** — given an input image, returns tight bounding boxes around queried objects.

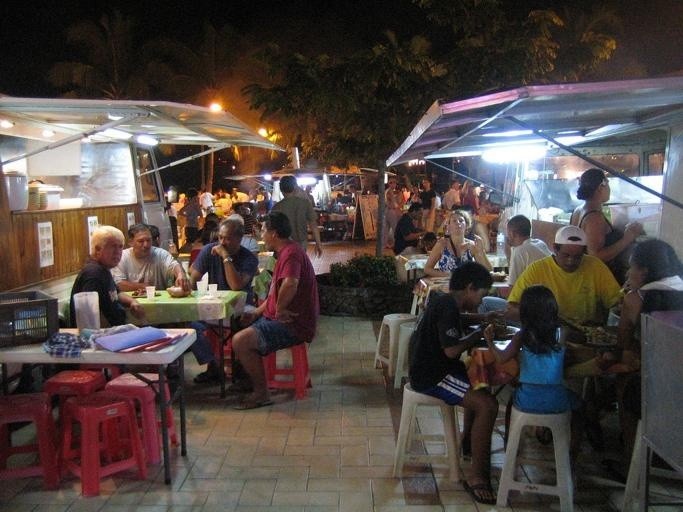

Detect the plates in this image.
[496,326,521,338]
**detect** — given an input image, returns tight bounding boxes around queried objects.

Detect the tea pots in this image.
[74,292,101,335]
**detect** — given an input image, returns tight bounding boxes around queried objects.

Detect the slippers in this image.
[226,379,274,411]
[461,480,496,506]
[194,369,226,385]
[535,427,553,447]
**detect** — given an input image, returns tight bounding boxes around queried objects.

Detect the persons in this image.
[70,173,321,411]
[384,168,682,505]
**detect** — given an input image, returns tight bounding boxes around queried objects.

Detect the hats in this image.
[555,226,589,247]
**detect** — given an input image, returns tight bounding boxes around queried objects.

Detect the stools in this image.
[204,301,312,400]
[394,322,418,389]
[0,364,180,497]
[496,406,576,512]
[390,383,462,483]
[374,313,417,378]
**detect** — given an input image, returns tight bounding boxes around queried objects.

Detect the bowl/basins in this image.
[28,187,49,210]
[491,276,505,281]
[63,198,83,209]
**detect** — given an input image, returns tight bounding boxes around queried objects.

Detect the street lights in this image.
[201,102,221,191]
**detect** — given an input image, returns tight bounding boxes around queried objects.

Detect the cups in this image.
[197,281,217,299]
[146,286,155,300]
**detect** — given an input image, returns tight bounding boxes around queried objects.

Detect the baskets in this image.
[0,289,59,349]
